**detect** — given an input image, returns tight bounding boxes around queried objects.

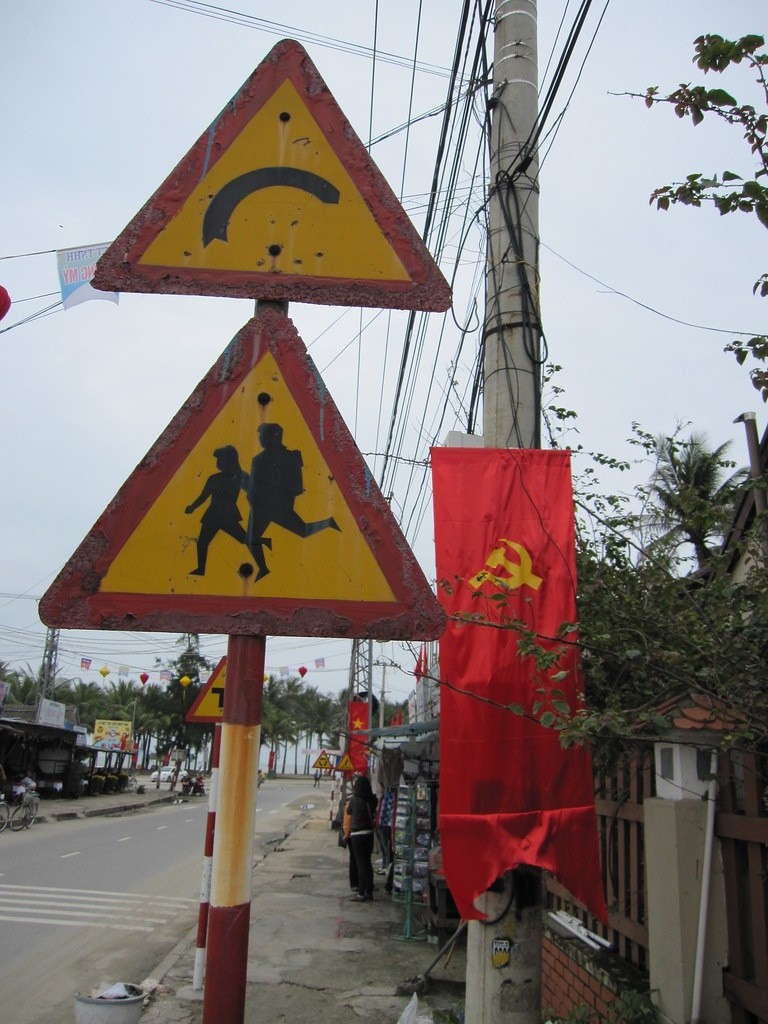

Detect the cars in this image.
[150,765,188,784]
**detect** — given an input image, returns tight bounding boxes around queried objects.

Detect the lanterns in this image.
[264,674,268,683]
[180,675,191,686]
[298,667,307,677]
[99,667,109,678]
[140,673,149,684]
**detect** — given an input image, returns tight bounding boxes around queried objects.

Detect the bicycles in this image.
[10,790,39,832]
[0,794,9,833]
[119,772,137,793]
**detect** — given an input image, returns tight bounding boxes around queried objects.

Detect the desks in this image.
[428,870,460,951]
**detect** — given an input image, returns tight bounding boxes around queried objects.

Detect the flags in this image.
[345,702,370,780]
[414,642,428,683]
[431,446,610,924]
[268,752,274,769]
[390,710,403,727]
[132,743,138,762]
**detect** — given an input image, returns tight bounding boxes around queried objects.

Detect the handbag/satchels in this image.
[338,831,347,849]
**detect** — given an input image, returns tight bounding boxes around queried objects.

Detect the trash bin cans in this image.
[71,981,145,1023]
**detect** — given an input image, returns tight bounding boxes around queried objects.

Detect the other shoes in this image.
[351,886,359,891]
[349,893,373,901]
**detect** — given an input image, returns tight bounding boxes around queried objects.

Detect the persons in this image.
[314,770,321,788]
[257,769,265,787]
[191,777,204,796]
[15,770,36,804]
[336,776,395,901]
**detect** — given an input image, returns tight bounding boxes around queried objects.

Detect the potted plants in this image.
[88,773,129,796]
